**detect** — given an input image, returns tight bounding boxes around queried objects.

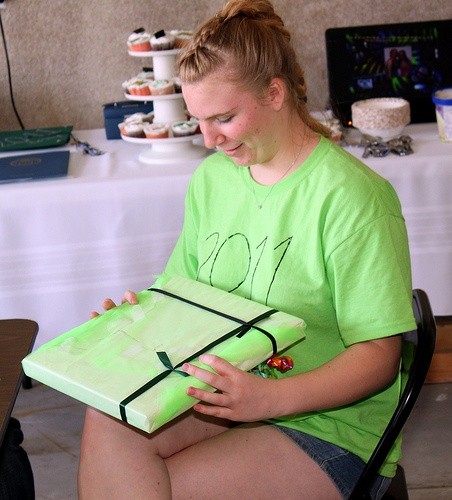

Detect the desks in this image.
[0,120,452,392]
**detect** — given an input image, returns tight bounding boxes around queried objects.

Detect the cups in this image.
[432,88,452,143]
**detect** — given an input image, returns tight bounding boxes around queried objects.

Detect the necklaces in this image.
[253,125,305,209]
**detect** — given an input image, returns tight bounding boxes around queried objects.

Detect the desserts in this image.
[122,66,184,95]
[126,27,196,51]
[117,109,202,139]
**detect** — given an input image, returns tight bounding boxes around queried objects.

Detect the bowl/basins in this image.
[351,98,411,141]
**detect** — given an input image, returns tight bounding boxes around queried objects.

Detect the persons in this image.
[386,49,411,76]
[78,0,417,500]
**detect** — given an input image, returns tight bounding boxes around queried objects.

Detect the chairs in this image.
[0,318,40,442]
[348,289,440,500]
